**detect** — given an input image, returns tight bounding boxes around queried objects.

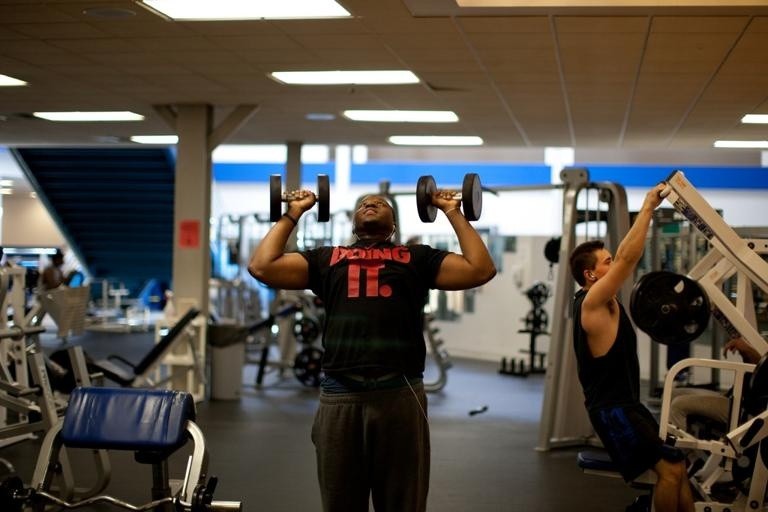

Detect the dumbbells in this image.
[417,173,482,222]
[269,174,330,222]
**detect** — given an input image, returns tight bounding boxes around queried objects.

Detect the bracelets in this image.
[283,212,297,225]
[445,206,460,215]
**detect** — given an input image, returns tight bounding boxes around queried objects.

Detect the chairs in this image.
[89,305,207,388]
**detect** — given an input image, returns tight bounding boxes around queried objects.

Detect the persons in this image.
[661,333,768,431]
[569,182,699,512]
[43,249,68,287]
[246,190,497,512]
